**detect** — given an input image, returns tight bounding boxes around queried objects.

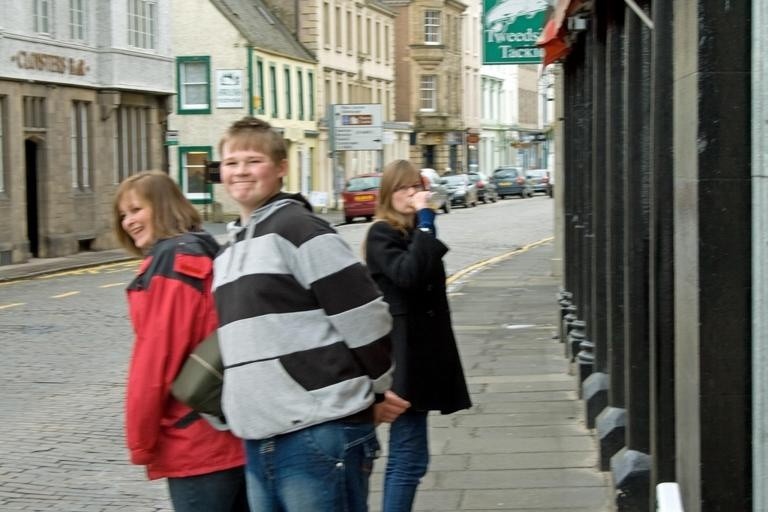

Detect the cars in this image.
[343,166,554,225]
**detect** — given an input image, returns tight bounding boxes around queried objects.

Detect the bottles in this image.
[409,191,442,209]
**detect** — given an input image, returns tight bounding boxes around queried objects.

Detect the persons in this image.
[110,166,250,511]
[361,156,474,510]
[207,115,413,510]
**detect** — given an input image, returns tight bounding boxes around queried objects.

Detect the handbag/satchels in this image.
[170,330,223,420]
